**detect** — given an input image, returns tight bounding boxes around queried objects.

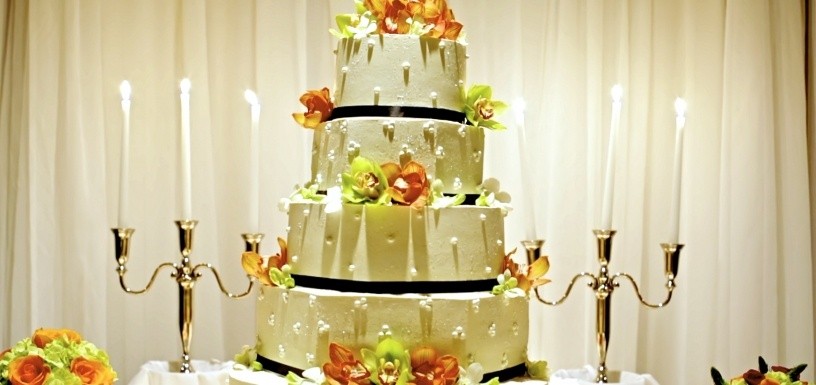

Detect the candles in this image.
[179,77,194,220]
[245,87,262,230]
[602,87,624,228]
[115,80,132,226]
[513,114,537,240]
[668,96,688,244]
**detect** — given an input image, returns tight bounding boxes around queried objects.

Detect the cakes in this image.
[0,0,810,385]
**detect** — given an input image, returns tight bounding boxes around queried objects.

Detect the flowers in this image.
[329,0,465,41]
[291,87,334,129]
[324,337,483,385]
[377,160,436,206]
[464,83,508,131]
[341,157,392,204]
[504,248,553,294]
[476,178,513,216]
[0,328,114,385]
